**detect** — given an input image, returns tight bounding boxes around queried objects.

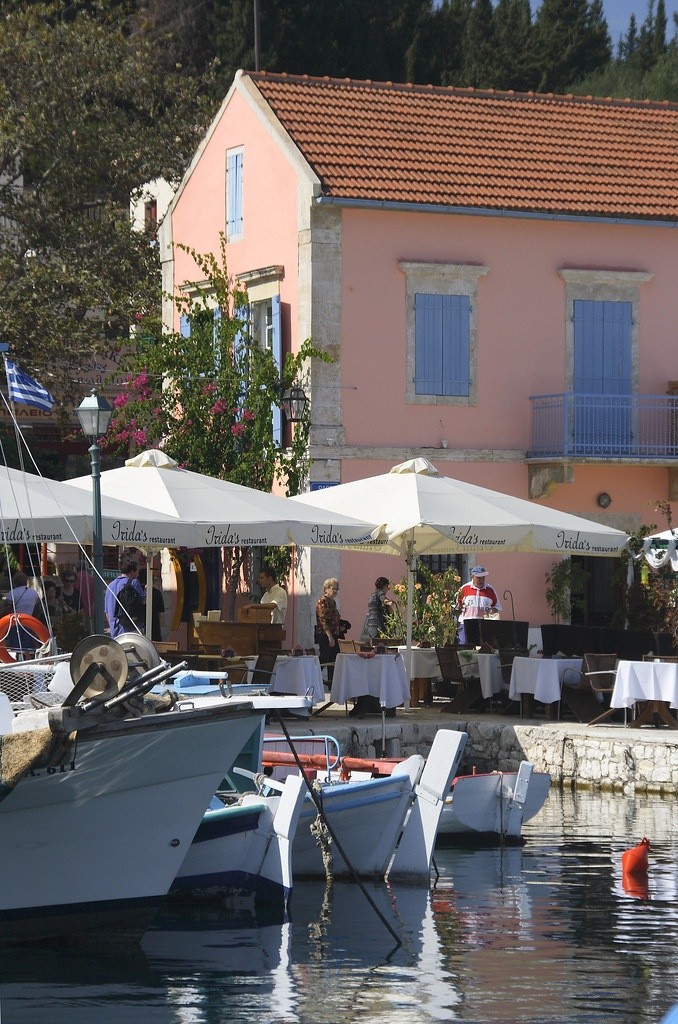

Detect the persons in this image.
[104,562,165,642]
[425,566,502,645]
[316,578,341,691]
[6,559,93,653]
[242,566,288,629]
[368,577,393,644]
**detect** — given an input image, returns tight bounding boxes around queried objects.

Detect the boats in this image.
[0,882,463,1024]
[145,667,552,904]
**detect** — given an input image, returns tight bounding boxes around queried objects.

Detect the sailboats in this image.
[0,392,317,934]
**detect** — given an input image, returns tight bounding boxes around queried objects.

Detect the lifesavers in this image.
[1,613,53,663]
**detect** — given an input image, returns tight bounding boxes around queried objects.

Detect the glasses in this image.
[64,578,75,583]
[328,587,339,591]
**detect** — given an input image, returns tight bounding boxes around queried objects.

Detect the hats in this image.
[471,565,489,578]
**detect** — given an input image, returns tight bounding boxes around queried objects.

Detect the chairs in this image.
[497,648,530,719]
[642,654,678,729]
[151,639,222,683]
[557,650,647,726]
[338,638,365,653]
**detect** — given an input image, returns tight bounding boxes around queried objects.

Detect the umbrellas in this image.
[285,458,628,710]
[0,465,200,545]
[59,449,389,644]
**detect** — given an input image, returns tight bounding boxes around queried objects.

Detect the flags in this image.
[7,361,55,412]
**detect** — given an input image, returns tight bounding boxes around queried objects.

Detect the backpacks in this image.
[116,578,146,630]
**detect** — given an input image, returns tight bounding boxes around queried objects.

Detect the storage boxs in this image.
[238,608,271,623]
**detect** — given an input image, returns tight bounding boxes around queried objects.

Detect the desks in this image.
[609,660,678,729]
[390,644,442,707]
[508,656,583,719]
[245,656,325,722]
[457,650,509,714]
[162,651,254,681]
[329,653,411,719]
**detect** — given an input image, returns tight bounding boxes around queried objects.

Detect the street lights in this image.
[73,389,113,635]
